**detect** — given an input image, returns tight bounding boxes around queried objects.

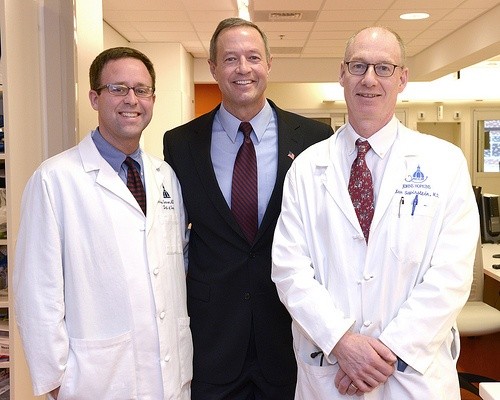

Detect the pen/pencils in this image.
[410,195,419,217]
[397,197,404,217]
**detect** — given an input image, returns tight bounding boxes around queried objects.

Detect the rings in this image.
[351,383,358,390]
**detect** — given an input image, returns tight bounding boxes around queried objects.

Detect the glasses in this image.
[343,61,403,77]
[94,83,154,98]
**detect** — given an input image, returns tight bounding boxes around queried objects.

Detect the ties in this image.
[348,139,375,245]
[231,122,258,249]
[123,157,147,217]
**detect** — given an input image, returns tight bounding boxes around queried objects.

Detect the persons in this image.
[163,0,335,400]
[13,48,193,400]
[271,26,480,400]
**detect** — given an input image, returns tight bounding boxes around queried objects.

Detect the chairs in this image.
[471,185,500,244]
[453,225,500,396]
